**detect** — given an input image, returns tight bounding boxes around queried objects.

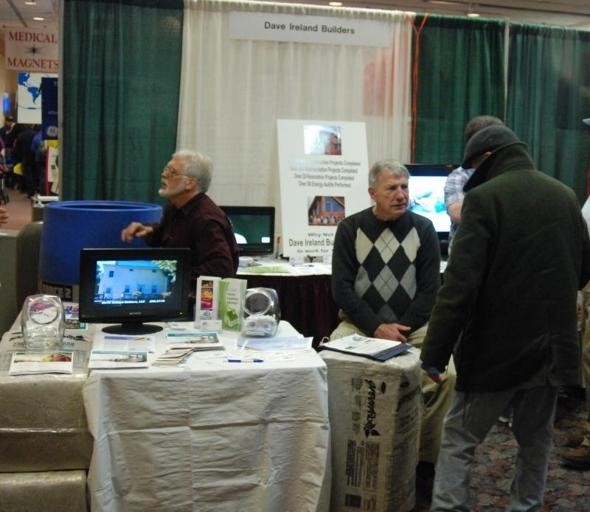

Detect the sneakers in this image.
[567,431,590,448]
[558,445,590,470]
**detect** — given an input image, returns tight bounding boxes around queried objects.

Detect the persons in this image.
[420,123,589,512]
[121,149,240,280]
[329,158,456,467]
[443,114,501,254]
[560,118,590,470]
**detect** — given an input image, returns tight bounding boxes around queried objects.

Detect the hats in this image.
[459,125,520,169]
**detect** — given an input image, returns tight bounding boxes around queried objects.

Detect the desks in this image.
[235,258,449,353]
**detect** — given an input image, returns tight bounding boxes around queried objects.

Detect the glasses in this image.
[163,166,191,179]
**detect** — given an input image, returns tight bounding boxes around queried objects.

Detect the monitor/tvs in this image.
[217,205,275,255]
[402,164,460,240]
[78,247,196,336]
